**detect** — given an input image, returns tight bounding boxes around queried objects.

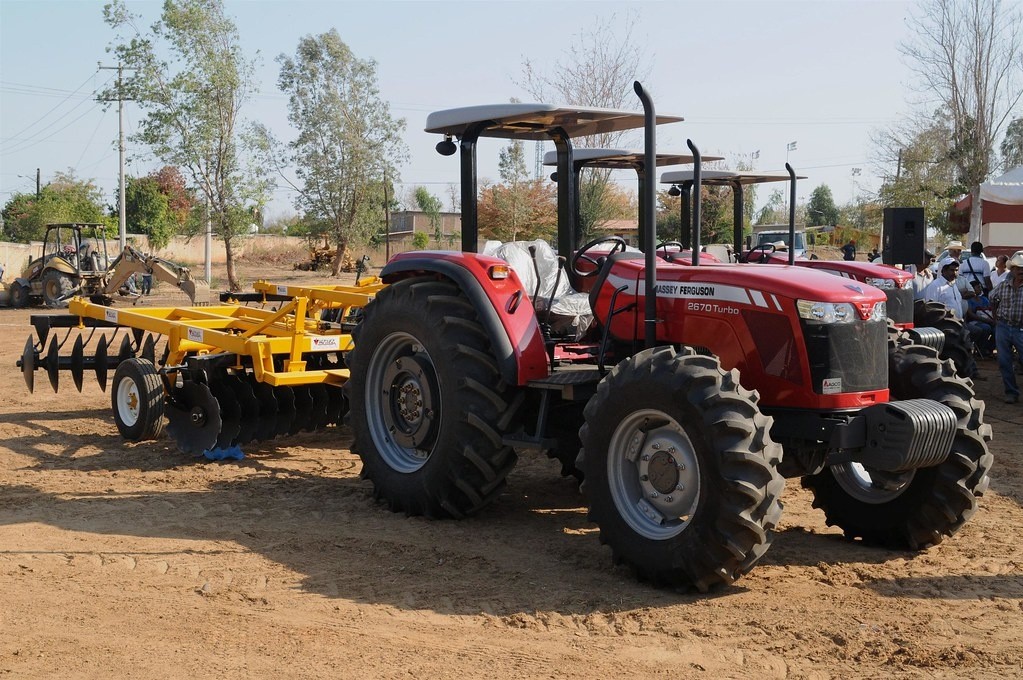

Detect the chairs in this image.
[701,244,740,264]
[495,239,596,344]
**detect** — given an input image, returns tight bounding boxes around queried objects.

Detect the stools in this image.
[969,338,987,360]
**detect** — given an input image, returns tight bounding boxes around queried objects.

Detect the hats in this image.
[938,257,961,274]
[1006,255,1023,270]
[944,241,966,250]
[771,240,789,251]
[850,240,856,245]
[925,249,936,258]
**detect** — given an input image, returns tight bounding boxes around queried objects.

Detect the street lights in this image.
[17,168,40,200]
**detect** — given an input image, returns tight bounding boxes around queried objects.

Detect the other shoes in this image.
[973,374,988,381]
[1004,393,1019,403]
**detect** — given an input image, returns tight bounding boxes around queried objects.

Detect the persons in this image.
[141,271,152,295]
[770,240,789,253]
[868,240,1023,403]
[840,240,856,261]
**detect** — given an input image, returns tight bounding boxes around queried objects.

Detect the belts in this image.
[1001,319,1020,326]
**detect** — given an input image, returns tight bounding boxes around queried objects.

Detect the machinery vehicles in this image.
[337,80,997,595]
[0,222,210,309]
[293,229,354,272]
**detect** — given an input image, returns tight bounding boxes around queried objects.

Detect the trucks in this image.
[752,222,807,260]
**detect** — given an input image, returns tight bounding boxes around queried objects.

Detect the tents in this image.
[944,164,1023,264]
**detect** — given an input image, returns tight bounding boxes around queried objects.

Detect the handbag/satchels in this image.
[975,306,993,320]
[983,288,991,297]
